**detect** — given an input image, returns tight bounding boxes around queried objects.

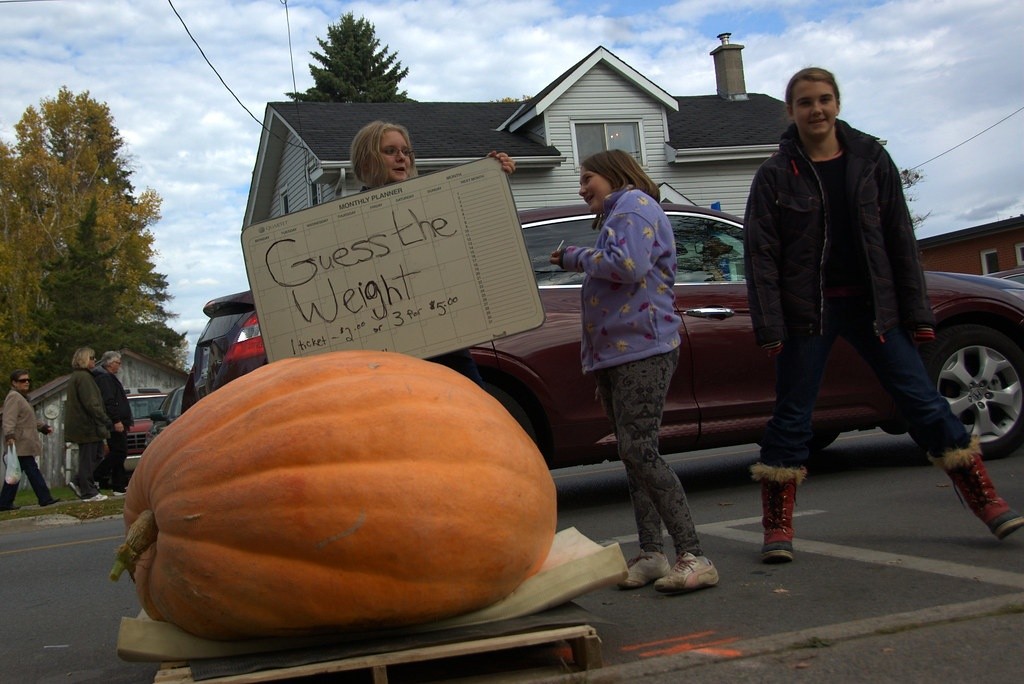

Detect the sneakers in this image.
[68,482,82,497]
[83,493,108,502]
[653,551,720,592]
[618,548,671,588]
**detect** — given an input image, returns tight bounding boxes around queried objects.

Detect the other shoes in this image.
[113,488,128,496]
[93,481,100,489]
[0,505,20,511]
[40,497,60,506]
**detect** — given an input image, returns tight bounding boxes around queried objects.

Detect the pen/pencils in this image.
[556,240,565,252]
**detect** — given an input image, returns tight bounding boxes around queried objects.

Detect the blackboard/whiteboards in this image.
[240,156,546,364]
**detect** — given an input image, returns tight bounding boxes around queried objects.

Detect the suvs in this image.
[182,204,1024,475]
[122,388,169,472]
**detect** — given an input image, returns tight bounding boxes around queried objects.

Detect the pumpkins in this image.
[108,350,557,638]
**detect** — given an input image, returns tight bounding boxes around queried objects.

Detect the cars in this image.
[144,385,186,449]
[980,266,1024,286]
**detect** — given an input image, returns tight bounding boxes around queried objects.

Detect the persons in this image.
[65,347,134,502]
[744,67,1024,562]
[0,371,61,511]
[549,149,719,589]
[351,119,516,394]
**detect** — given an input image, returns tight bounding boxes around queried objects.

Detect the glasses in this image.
[378,146,414,159]
[114,360,121,366]
[19,379,33,384]
[89,356,95,360]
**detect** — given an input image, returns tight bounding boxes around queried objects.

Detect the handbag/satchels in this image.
[3,443,21,484]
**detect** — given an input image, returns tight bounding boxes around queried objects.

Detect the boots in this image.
[750,461,805,563]
[926,435,1024,541]
